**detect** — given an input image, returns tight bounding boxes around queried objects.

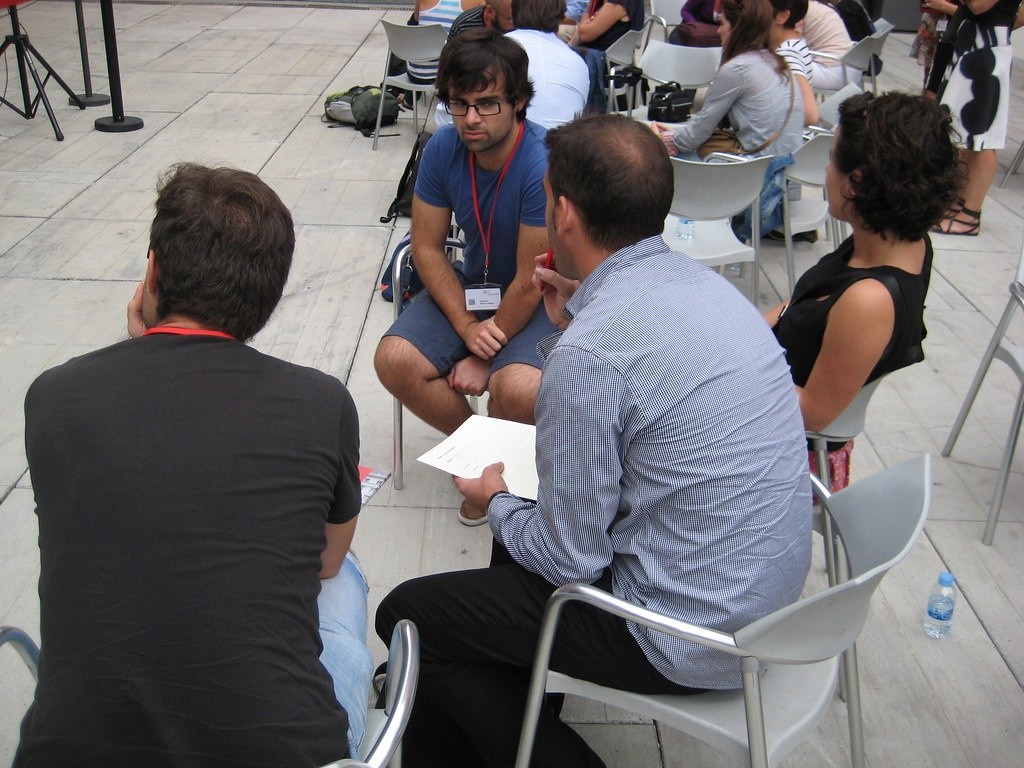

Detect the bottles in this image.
[676,217,693,239]
[729,261,742,277]
[923,572,956,638]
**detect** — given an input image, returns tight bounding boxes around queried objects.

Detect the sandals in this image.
[932,207,981,236]
[931,197,965,223]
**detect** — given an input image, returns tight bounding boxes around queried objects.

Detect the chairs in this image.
[719,435,721,437]
[371,18,448,151]
[609,0,897,308]
[804,370,886,768]
[390,235,467,491]
[513,450,931,768]
[998,140,1024,189]
[0,619,422,768]
[940,241,1024,547]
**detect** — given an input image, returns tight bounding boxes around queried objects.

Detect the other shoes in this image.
[457,500,488,526]
[398,100,413,110]
[395,94,405,103]
[768,227,817,244]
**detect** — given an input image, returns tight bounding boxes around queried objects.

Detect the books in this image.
[358,466,391,510]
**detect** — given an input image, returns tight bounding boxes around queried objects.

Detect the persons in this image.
[406,0,486,85]
[650,0,806,164]
[375,115,813,767]
[761,90,970,507]
[500,0,590,130]
[922,0,1022,236]
[374,28,572,526]
[559,0,590,25]
[11,162,375,768]
[817,0,883,77]
[447,0,515,43]
[794,0,863,90]
[573,0,645,89]
[769,0,819,126]
[669,0,723,109]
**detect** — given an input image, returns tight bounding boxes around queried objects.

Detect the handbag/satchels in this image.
[648,81,693,123]
[678,21,721,47]
[698,130,739,157]
[321,85,401,137]
[379,231,423,302]
[380,131,433,223]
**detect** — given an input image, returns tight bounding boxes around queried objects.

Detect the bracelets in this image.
[485,490,510,515]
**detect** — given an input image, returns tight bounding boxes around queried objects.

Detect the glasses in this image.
[445,95,511,116]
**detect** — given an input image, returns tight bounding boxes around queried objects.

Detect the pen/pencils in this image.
[540,247,553,293]
